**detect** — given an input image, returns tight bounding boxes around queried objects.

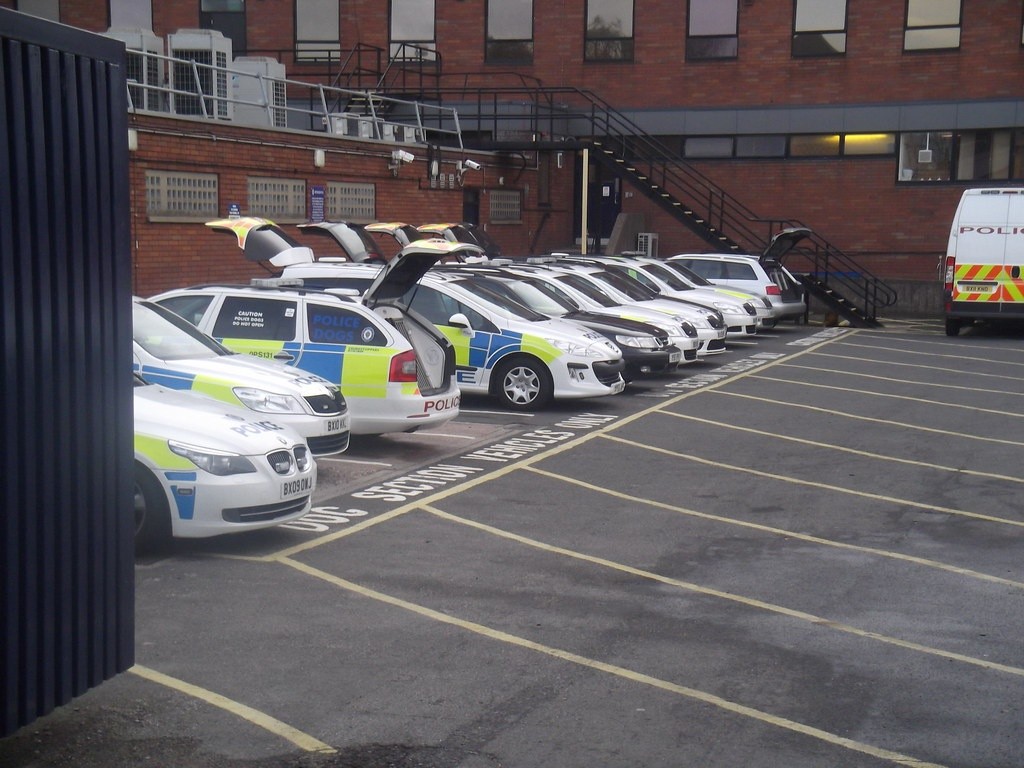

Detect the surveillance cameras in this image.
[465,160,480,170]
[396,149,415,163]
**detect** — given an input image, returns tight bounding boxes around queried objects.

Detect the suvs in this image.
[204,216,627,413]
[416,224,729,356]
[364,222,699,364]
[295,221,682,379]
[146,238,487,441]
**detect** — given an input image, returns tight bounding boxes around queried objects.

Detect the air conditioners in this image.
[332,113,360,137]
[361,117,385,140]
[407,128,427,144]
[385,124,407,141]
[637,232,659,258]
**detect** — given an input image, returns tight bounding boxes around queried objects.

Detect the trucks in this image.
[943,188,1024,336]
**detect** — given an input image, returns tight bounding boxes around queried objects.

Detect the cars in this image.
[564,255,759,337]
[133,295,350,457]
[668,226,813,324]
[630,256,777,330]
[133,372,319,557]
